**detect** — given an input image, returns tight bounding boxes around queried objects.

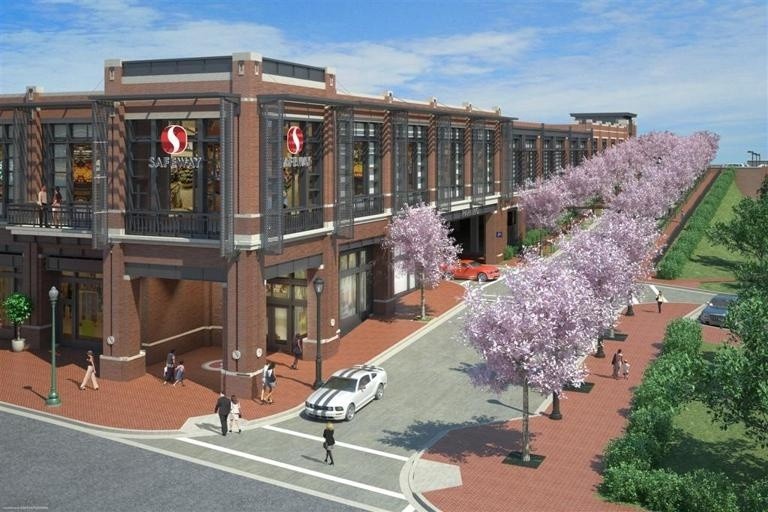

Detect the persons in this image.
[214,390,243,436]
[164,353,184,386]
[260,362,278,403]
[323,422,335,465]
[38,184,62,228]
[612,349,630,380]
[656,291,662,313]
[292,334,303,370]
[80,351,99,390]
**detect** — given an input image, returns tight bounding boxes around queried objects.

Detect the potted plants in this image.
[0,291,35,353]
